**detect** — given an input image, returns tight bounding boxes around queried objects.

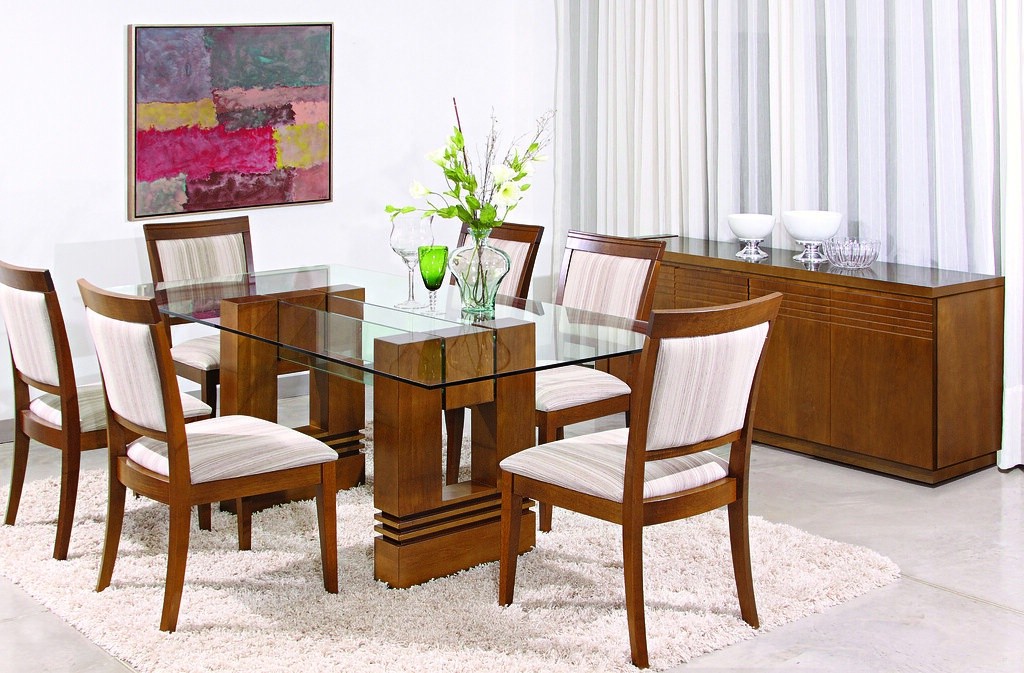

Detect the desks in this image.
[95,266,668,587]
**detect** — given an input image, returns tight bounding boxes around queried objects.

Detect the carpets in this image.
[2,452,900,671]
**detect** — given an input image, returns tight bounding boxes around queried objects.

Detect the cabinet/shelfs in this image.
[632,235,1006,489]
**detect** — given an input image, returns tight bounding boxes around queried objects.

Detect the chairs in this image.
[447,219,545,310]
[498,288,782,668]
[556,230,666,320]
[78,277,347,630]
[1,261,213,559]
[143,216,256,291]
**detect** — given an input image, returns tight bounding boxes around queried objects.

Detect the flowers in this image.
[386,105,564,245]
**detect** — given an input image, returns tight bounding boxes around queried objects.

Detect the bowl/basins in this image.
[726,213,776,239]
[779,210,843,240]
[822,236,881,268]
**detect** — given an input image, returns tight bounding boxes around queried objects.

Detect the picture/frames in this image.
[125,19,335,221]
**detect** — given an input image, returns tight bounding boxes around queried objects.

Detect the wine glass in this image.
[418,246,449,317]
[391,211,433,309]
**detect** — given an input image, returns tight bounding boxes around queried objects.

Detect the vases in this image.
[450,227,512,319]
